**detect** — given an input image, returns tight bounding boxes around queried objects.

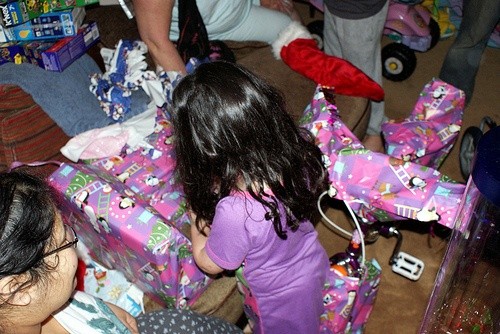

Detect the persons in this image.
[172,61,330,334]
[135,0,322,80]
[0,171,245,334]
[324,0,389,154]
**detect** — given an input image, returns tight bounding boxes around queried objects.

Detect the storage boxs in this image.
[43,161,215,311]
[0,0,102,72]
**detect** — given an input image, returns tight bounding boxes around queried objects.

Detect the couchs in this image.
[0,5,370,323]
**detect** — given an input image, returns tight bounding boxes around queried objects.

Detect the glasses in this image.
[6,223,79,276]
